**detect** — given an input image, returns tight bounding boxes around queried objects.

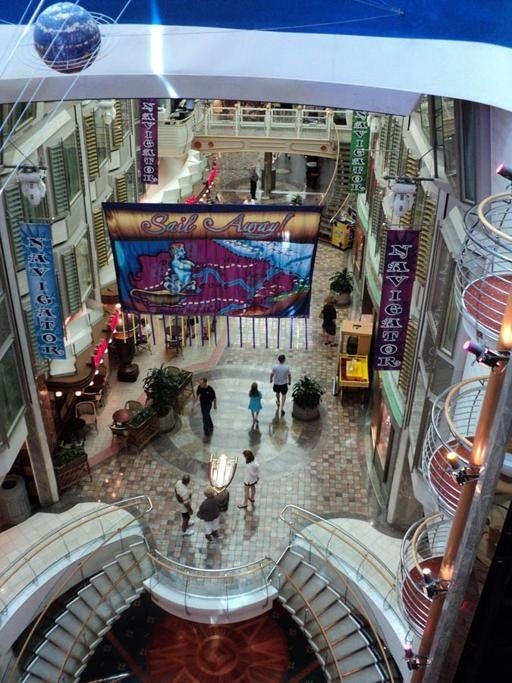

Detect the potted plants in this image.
[127,404,160,453]
[291,375,325,421]
[144,362,182,432]
[326,262,353,306]
[51,444,93,492]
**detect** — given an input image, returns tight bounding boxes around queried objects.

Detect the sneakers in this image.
[276,400,281,407]
[180,529,194,536]
[187,521,194,526]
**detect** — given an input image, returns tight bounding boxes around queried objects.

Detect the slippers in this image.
[237,504,247,508]
[248,497,255,503]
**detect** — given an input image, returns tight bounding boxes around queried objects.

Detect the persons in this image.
[196,485,225,545]
[269,354,291,416]
[236,449,260,509]
[247,382,262,424]
[242,194,256,206]
[319,296,338,348]
[248,169,258,200]
[213,483,229,533]
[193,376,217,443]
[173,475,194,536]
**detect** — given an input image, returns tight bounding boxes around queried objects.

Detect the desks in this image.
[111,407,132,426]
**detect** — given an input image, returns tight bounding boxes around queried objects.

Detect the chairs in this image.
[123,398,142,412]
[61,365,108,444]
[134,314,215,356]
[108,424,129,447]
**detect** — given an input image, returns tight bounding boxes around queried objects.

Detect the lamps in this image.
[442,449,481,485]
[401,643,432,670]
[418,564,452,599]
[458,333,512,370]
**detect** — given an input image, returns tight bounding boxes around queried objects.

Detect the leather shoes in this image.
[212,530,219,537]
[206,534,213,541]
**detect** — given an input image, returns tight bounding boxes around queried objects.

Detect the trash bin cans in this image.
[0,474,32,525]
[261,167,276,191]
